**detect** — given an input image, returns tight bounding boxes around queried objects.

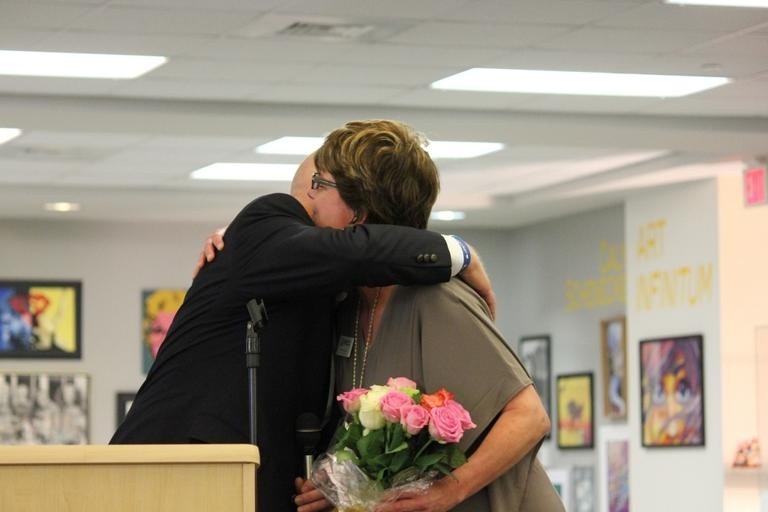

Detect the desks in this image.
[1,443,260,512]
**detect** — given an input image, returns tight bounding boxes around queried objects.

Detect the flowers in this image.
[325,376,476,491]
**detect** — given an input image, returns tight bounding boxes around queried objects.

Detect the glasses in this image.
[312,173,337,190]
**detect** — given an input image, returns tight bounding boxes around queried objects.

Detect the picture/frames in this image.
[116,391,136,427]
[141,288,197,373]
[555,373,594,450]
[0,370,91,446]
[599,315,628,422]
[516,337,551,442]
[2,282,81,359]
[638,334,704,448]
[569,439,629,512]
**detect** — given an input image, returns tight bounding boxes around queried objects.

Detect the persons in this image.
[0,374,85,444]
[192,118,568,511]
[108,151,497,510]
